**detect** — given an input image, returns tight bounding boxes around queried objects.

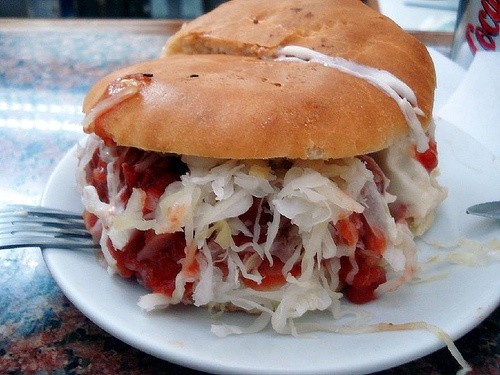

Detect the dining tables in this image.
[0,17,500,375]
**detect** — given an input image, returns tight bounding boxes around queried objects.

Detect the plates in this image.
[40,114,500,374]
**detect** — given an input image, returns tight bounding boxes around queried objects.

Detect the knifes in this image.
[465,201,500,218]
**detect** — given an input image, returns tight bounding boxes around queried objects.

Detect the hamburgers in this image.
[82,1,447,336]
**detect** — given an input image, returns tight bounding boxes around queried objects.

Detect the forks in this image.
[0,204,100,250]
